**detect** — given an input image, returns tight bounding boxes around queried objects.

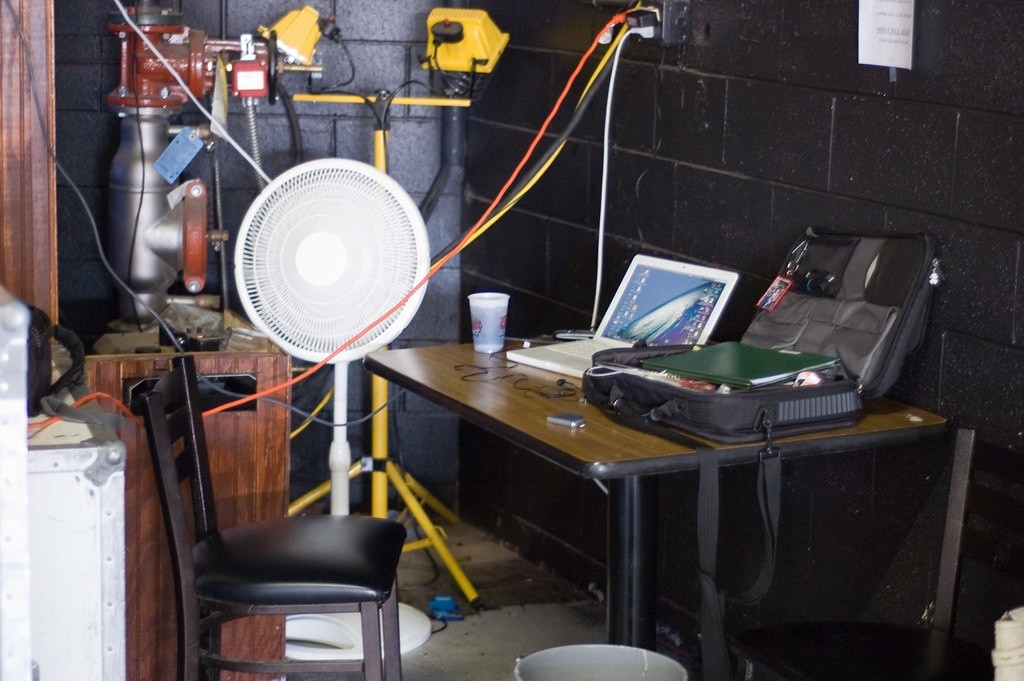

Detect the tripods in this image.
[284,107,488,613]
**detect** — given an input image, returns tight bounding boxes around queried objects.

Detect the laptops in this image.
[507,254,739,378]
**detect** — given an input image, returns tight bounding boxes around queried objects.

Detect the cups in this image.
[468,292,510,353]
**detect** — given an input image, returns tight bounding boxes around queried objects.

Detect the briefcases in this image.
[577,223,937,441]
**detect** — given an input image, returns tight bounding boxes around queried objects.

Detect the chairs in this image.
[141,355,407,681]
[732,428,1024,681]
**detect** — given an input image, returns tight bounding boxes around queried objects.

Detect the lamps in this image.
[257,5,330,69]
[417,4,511,75]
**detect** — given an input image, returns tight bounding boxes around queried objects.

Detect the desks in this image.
[25,344,128,681]
[362,335,951,650]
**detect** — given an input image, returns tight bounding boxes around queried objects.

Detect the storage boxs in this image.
[577,228,943,446]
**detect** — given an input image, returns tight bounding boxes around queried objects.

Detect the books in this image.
[639,341,839,390]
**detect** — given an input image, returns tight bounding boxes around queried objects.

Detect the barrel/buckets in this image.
[514,644,688,681]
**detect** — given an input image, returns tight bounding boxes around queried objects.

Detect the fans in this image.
[230,157,434,663]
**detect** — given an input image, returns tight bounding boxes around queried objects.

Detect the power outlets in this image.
[631,0,691,44]
